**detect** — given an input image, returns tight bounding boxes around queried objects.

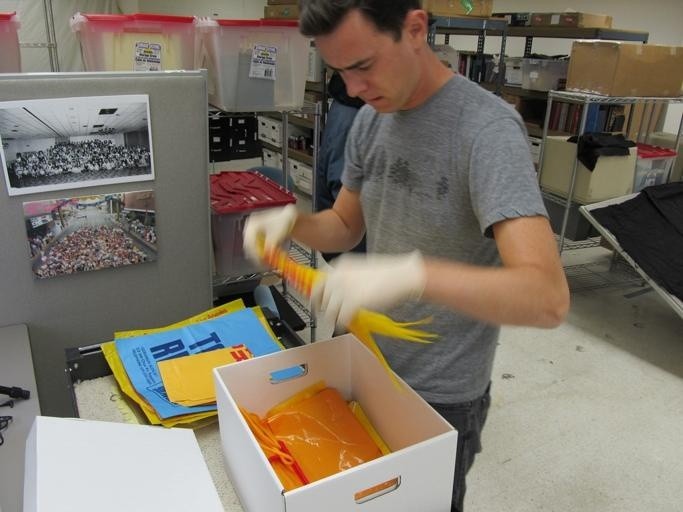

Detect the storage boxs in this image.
[212,332,461,512]
[23,416,225,512]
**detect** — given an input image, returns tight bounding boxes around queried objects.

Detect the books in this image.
[550,100,583,134]
[456,50,507,84]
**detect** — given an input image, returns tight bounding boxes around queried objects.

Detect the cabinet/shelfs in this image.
[0,0,683,344]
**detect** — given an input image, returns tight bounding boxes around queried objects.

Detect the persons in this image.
[240,0,571,511]
[315,70,365,261]
[30,219,157,279]
[95,203,100,212]
[15,138,151,180]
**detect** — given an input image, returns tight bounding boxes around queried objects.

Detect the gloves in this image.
[244,204,299,271]
[307,250,427,334]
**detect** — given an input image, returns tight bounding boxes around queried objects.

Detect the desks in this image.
[72,373,246,512]
[0,323,44,512]
